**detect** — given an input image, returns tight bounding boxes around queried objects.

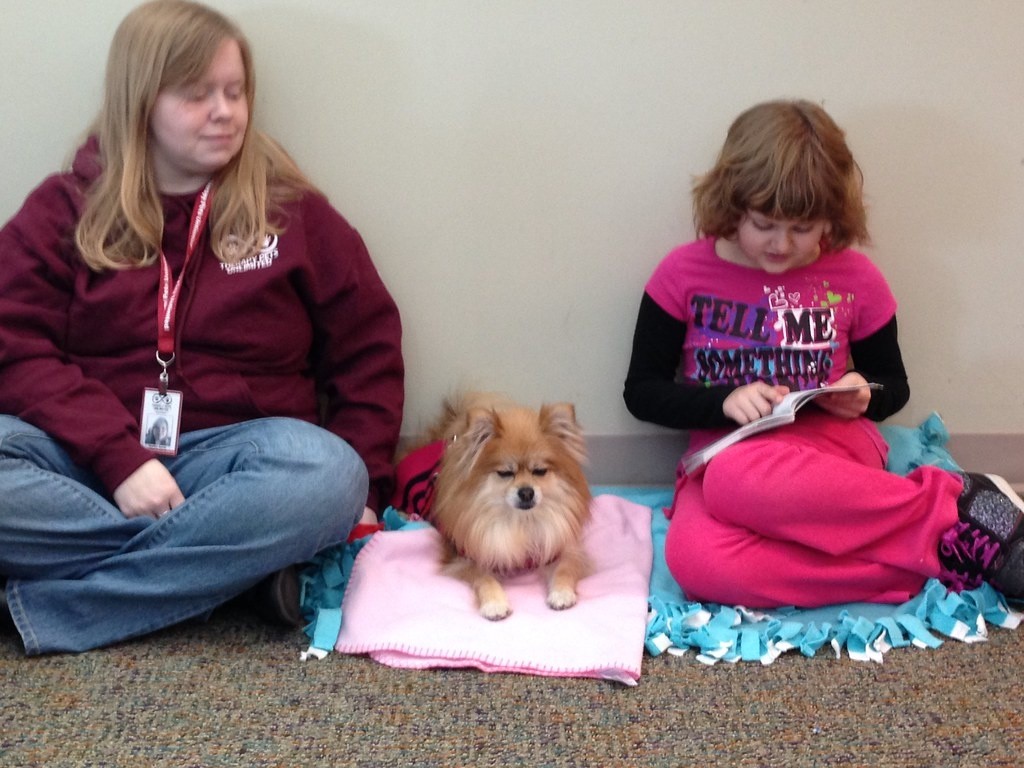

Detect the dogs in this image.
[399,394,594,620]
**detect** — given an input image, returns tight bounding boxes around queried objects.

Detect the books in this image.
[683,383,880,475]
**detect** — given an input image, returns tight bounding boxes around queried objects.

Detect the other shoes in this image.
[223,566,300,629]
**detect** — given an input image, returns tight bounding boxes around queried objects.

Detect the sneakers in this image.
[940,565,983,593]
[938,472,1024,600]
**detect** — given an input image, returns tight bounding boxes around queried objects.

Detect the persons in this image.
[624,100,1024,610]
[0,0,405,655]
[147,418,168,444]
